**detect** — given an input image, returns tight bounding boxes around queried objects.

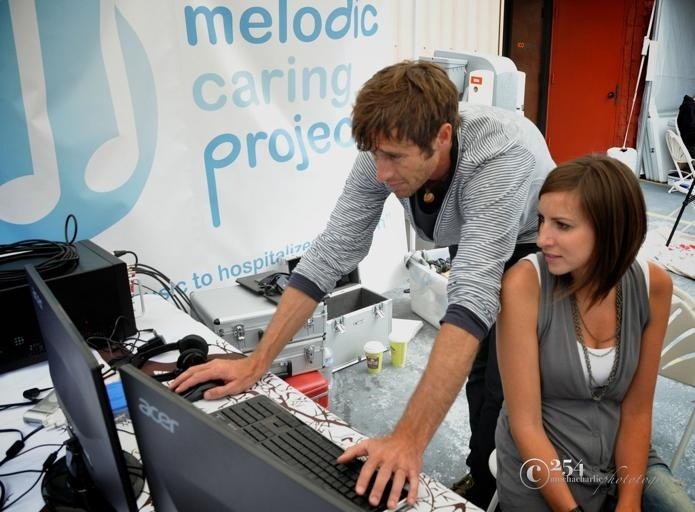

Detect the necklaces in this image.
[571,275,623,403]
[572,295,623,347]
[422,175,448,203]
[576,334,618,357]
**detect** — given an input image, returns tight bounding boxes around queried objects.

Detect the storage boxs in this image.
[408,247,451,328]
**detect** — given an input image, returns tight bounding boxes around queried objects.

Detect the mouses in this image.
[180,378,225,403]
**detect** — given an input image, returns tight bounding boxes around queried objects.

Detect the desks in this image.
[0,294,487,511]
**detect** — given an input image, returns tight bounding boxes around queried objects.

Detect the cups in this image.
[364,333,407,374]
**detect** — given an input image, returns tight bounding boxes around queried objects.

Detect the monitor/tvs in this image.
[119,362,365,512]
[22,263,146,512]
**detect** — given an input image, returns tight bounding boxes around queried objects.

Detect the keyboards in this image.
[207,394,408,512]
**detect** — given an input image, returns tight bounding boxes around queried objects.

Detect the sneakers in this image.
[452,474,496,506]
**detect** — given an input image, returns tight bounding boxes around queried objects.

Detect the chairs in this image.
[487,285,695,512]
[665,130,695,194]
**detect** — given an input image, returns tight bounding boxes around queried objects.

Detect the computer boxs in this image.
[0,239,137,375]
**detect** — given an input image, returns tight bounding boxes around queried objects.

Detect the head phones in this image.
[128,334,208,382]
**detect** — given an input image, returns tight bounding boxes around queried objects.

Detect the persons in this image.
[488,153,693,512]
[171,65,562,510]
